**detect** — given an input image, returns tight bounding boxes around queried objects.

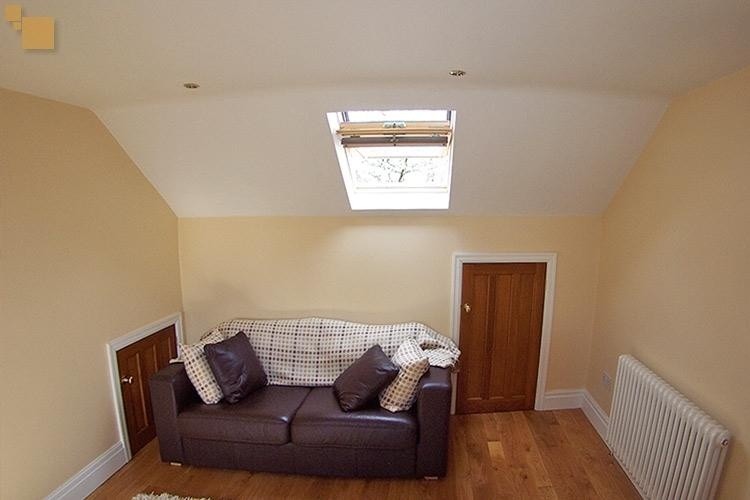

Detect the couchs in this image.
[148,316,453,481]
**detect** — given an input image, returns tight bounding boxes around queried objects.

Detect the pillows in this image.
[204,331,268,404]
[177,328,226,405]
[333,344,400,413]
[377,338,430,413]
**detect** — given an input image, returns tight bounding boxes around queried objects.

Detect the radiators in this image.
[605,354,731,500]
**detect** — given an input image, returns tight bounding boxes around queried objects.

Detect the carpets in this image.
[131,491,213,500]
